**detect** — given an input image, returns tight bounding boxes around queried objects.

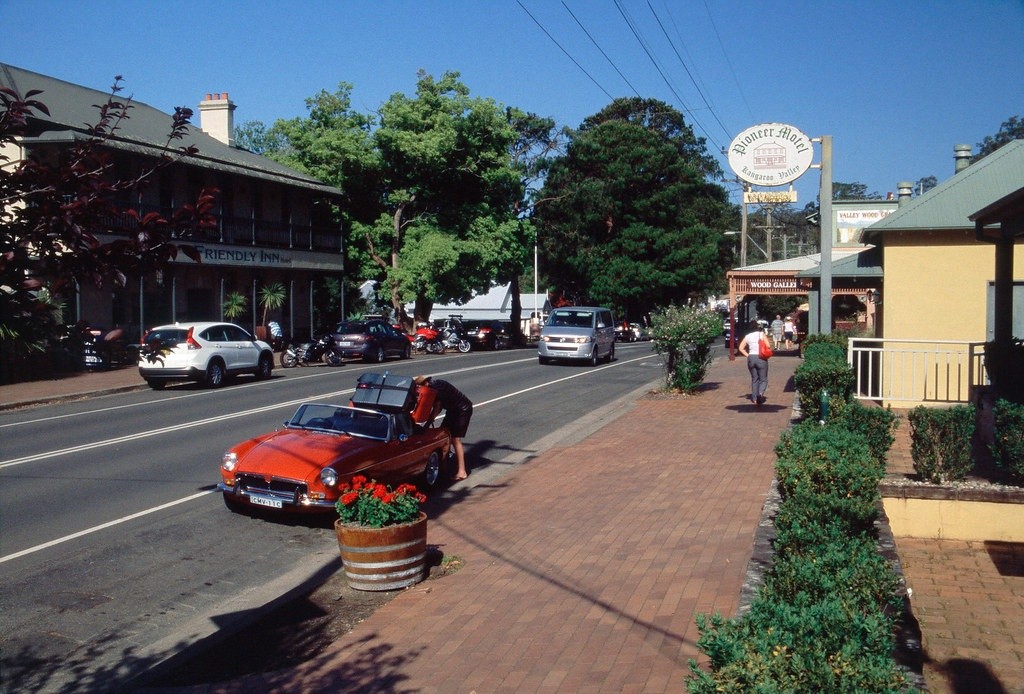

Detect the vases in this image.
[335,511,428,591]
[667,344,706,381]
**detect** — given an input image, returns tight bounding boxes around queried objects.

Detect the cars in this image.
[138,322,273,389]
[217,371,452,513]
[461,320,515,351]
[614,321,643,342]
[333,315,411,363]
[722,317,751,349]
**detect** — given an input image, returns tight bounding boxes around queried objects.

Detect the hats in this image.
[415,375,432,386]
[787,317,791,320]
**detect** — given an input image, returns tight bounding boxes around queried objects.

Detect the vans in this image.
[538,306,616,366]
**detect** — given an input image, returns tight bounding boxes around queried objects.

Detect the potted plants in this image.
[256,280,288,340]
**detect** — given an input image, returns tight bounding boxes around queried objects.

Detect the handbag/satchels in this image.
[758,339,773,360]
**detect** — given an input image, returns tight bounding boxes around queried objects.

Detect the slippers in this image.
[449,474,467,482]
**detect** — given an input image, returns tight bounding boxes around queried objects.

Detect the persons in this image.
[771,315,794,351]
[268,320,283,353]
[414,376,474,481]
[739,320,770,404]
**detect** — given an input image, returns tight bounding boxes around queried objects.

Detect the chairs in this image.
[76,339,128,370]
[326,407,360,432]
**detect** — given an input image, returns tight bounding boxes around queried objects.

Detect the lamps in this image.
[806,210,819,226]
[866,289,882,306]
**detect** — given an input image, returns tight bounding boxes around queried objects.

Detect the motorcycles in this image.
[280,331,342,368]
[392,320,472,354]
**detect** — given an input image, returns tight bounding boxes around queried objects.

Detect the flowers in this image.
[335,475,427,529]
[639,296,726,354]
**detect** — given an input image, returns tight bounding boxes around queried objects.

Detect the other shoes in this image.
[753,394,763,405]
[775,346,780,350]
